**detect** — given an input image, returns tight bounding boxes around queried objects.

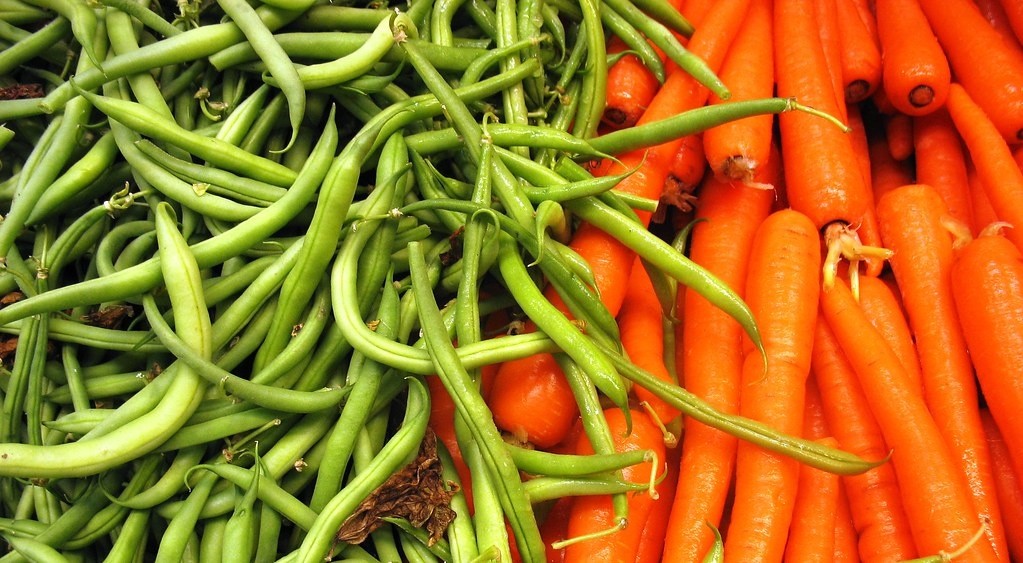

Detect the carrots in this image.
[420,0,1023,563]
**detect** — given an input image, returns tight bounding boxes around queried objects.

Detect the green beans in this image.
[0,0,896,563]
